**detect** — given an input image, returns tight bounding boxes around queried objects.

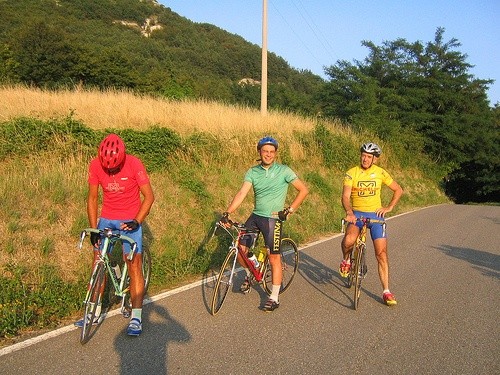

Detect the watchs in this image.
[288,206,295,212]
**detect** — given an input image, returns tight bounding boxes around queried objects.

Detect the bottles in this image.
[111,261,122,279]
[247,252,259,268]
[257,246,267,262]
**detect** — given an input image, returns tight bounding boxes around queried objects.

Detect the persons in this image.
[74,133,154,336]
[222,136,308,313]
[339,142,403,306]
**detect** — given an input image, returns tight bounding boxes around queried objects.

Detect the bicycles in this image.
[340,216,388,311]
[78,228,152,345]
[210,218,300,317]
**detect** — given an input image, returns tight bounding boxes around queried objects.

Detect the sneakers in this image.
[240,275,253,291]
[383,292,397,306]
[73,311,99,327]
[261,298,280,313]
[339,259,352,278]
[127,317,143,336]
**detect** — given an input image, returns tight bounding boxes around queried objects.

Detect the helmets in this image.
[360,142,382,158]
[97,134,126,171]
[257,136,278,150]
